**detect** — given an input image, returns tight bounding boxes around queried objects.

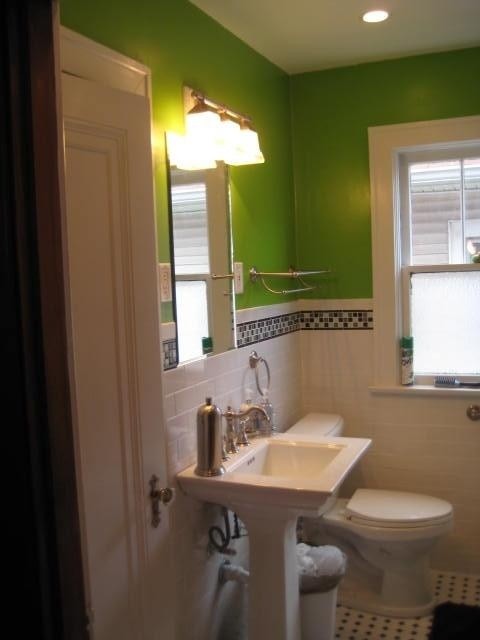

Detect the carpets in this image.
[429,601,480,640]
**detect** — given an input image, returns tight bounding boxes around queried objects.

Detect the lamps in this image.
[182,90,265,166]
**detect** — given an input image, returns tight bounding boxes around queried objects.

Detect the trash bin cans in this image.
[299,574,340,640]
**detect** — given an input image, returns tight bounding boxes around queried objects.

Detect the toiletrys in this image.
[257,391,273,439]
[240,388,260,436]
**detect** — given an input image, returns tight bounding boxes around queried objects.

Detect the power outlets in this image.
[233,263,243,296]
[159,263,173,302]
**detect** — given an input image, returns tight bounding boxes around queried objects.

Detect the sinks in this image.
[176,433,376,515]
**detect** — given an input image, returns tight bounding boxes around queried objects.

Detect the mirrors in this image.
[165,133,238,369]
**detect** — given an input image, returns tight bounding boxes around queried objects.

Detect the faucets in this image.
[224,404,269,453]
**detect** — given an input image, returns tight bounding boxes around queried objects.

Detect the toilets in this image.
[285,413,454,619]
